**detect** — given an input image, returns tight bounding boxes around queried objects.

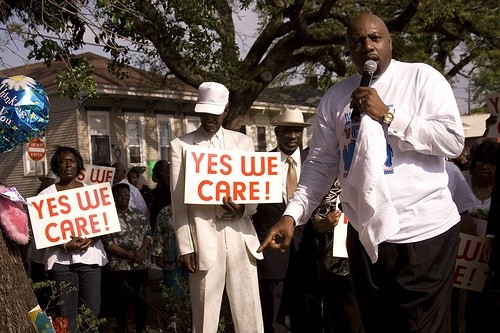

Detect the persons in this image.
[22,145,225,333]
[258,14,464,333]
[168,81,266,333]
[312,177,356,333]
[251,107,325,333]
[453,141,500,333]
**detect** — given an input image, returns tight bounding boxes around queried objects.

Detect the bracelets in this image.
[63,243,68,254]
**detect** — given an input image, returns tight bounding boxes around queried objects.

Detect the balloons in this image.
[0,75,49,154]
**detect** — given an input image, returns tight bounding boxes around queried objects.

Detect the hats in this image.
[194,82,230,115]
[269,107,313,127]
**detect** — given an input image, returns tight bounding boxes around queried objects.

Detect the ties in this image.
[286,156,297,201]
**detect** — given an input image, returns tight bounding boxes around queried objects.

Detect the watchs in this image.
[381,106,395,125]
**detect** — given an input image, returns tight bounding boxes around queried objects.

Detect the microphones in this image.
[350,60,377,123]
